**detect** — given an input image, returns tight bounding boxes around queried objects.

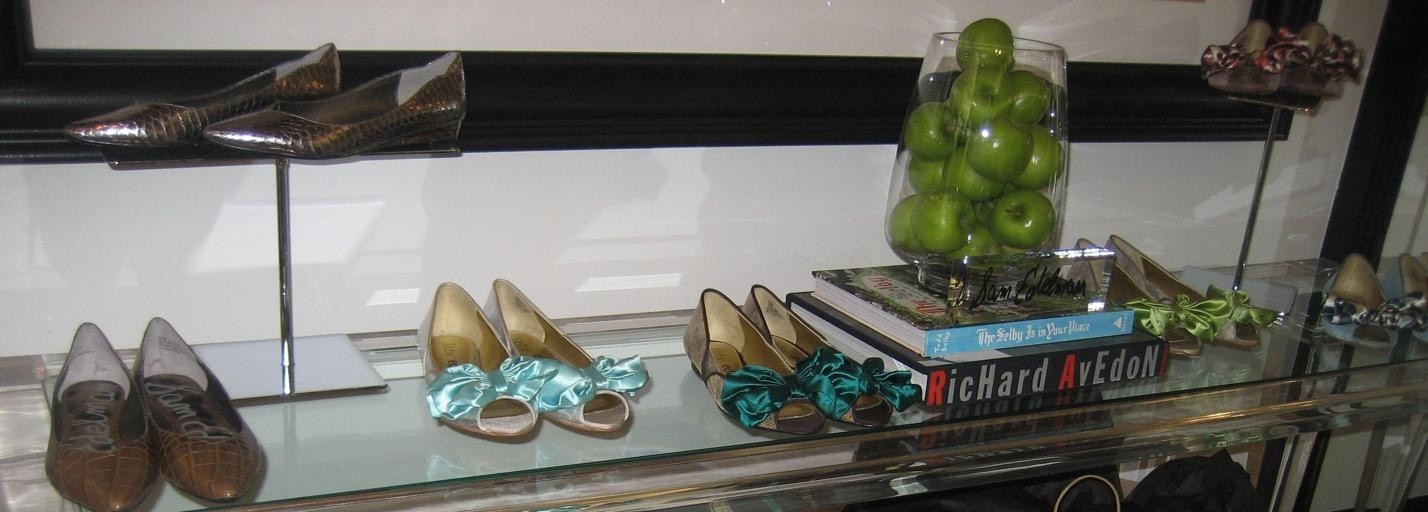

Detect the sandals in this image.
[680,288,824,437]
[140,317,268,503]
[1066,232,1283,359]
[414,280,539,437]
[1321,250,1426,352]
[205,48,472,162]
[481,277,658,436]
[742,284,924,426]
[66,43,342,150]
[45,321,160,510]
[1203,16,1367,102]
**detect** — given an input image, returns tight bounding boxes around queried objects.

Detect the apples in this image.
[886,69,1066,279]
[956,20,1015,73]
